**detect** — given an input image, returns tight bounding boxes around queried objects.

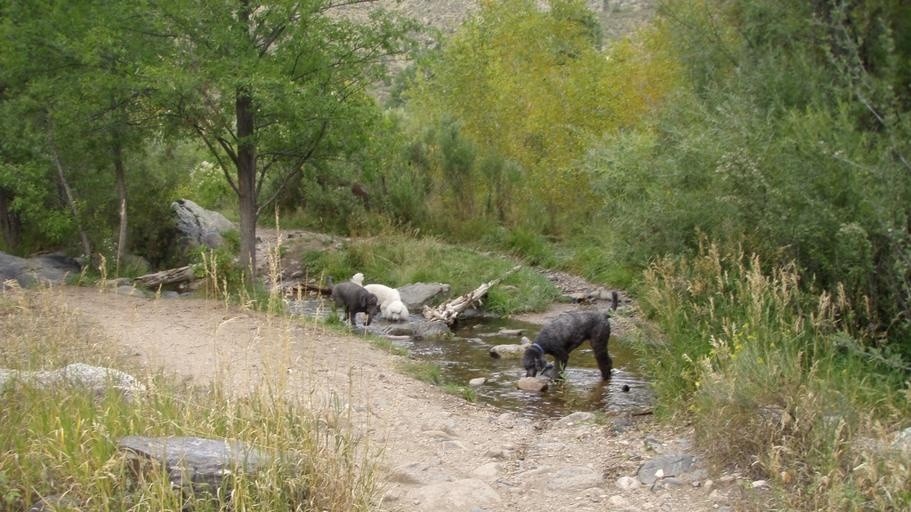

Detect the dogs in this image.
[329,282,381,328]
[349,272,409,322]
[522,291,619,380]
